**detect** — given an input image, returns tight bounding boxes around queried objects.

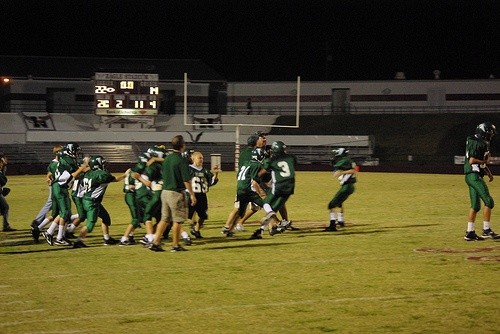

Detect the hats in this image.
[255,131,269,137]
[247,136,259,147]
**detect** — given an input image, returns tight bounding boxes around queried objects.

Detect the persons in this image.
[327,148,357,232]
[29,143,120,248]
[465,121,500,241]
[119,134,219,254]
[220,133,295,239]
[0,155,18,231]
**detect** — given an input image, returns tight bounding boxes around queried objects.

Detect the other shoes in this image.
[481,228,500,240]
[221,218,300,238]
[170,244,189,252]
[148,243,166,252]
[463,229,485,241]
[103,237,120,245]
[73,241,87,248]
[31,219,77,246]
[120,221,203,246]
[322,221,344,231]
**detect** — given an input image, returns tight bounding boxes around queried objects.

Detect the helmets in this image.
[89,155,106,171]
[58,147,76,159]
[476,122,496,142]
[271,141,287,156]
[139,144,180,163]
[64,143,82,158]
[331,147,349,164]
